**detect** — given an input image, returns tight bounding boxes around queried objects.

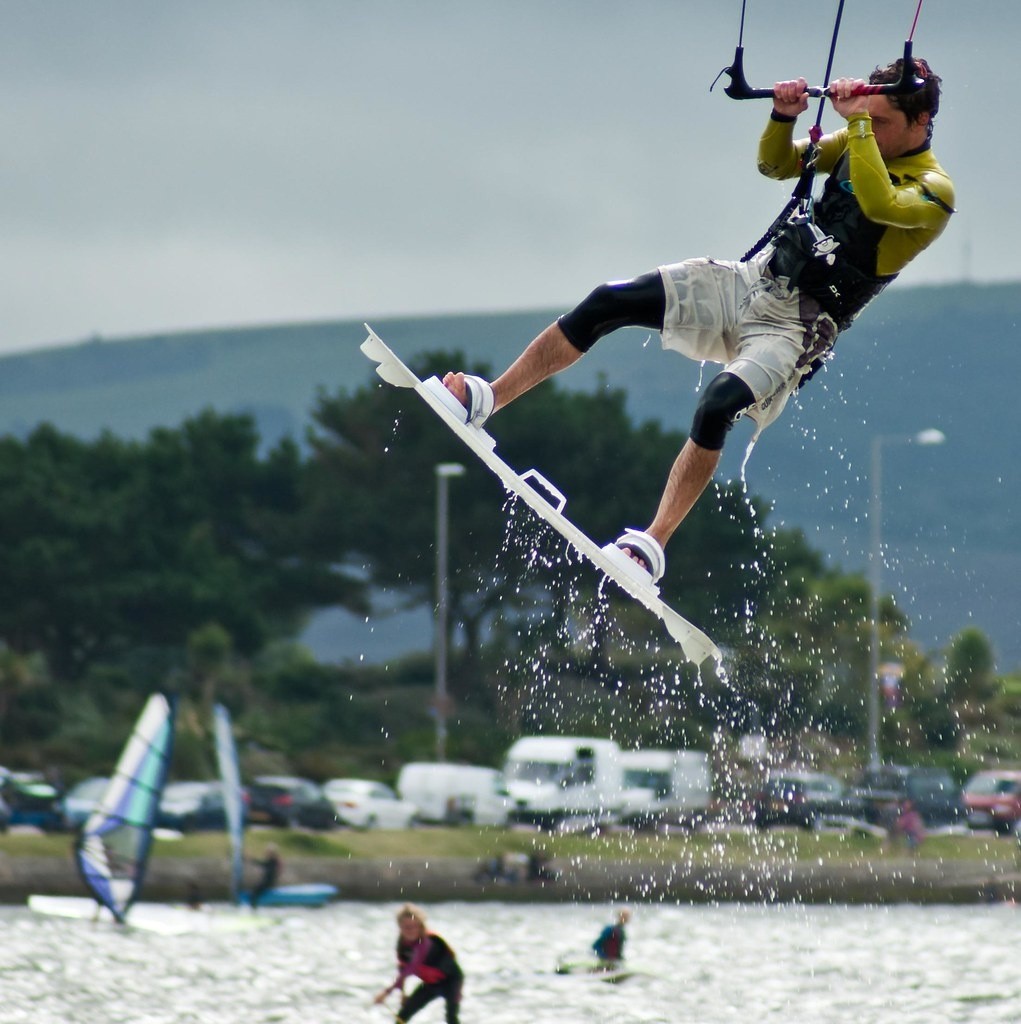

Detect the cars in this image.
[754,771,866,833]
[323,776,421,831]
[62,776,113,834]
[154,782,229,836]
[855,765,966,828]
[243,774,338,833]
[965,771,1021,839]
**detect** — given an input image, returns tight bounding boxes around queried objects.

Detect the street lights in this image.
[436,462,465,764]
[867,430,946,771]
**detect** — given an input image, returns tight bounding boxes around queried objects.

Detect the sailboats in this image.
[81,691,172,933]
[213,702,339,910]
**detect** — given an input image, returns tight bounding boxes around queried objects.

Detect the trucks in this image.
[401,731,714,834]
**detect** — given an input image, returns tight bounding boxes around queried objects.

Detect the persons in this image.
[591,909,630,963]
[370,905,466,1024]
[240,841,281,911]
[438,56,957,577]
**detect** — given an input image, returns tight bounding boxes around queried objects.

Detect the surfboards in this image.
[355,317,729,671]
